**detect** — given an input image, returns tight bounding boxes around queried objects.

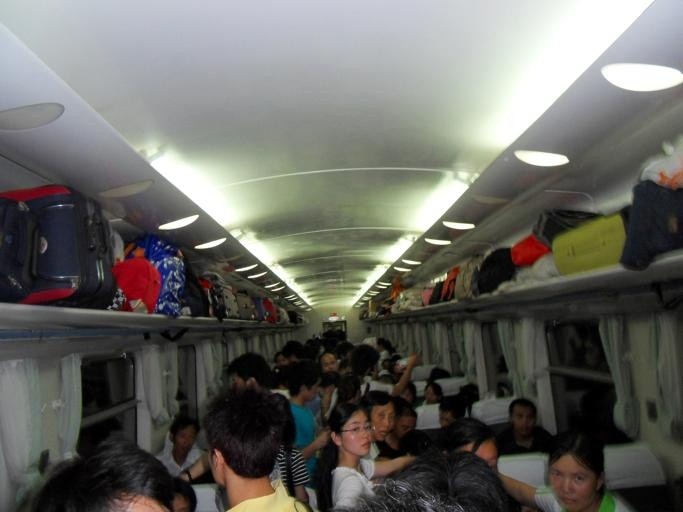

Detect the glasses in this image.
[339,423,376,434]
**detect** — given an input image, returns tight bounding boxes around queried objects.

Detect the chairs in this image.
[497,453,549,511]
[378,360,390,376]
[602,442,667,510]
[411,364,516,436]
[191,483,225,511]
